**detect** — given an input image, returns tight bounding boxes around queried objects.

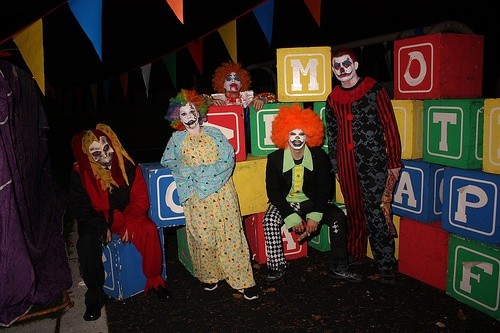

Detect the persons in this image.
[161,90,259,300]
[206,63,276,111]
[261,107,361,281]
[69,124,175,322]
[324,48,403,297]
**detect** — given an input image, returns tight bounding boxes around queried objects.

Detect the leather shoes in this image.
[159,291,174,302]
[83,302,105,321]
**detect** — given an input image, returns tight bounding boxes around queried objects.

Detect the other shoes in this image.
[382,273,395,287]
[335,269,357,279]
[261,271,286,288]
[238,288,260,301]
[203,283,218,294]
